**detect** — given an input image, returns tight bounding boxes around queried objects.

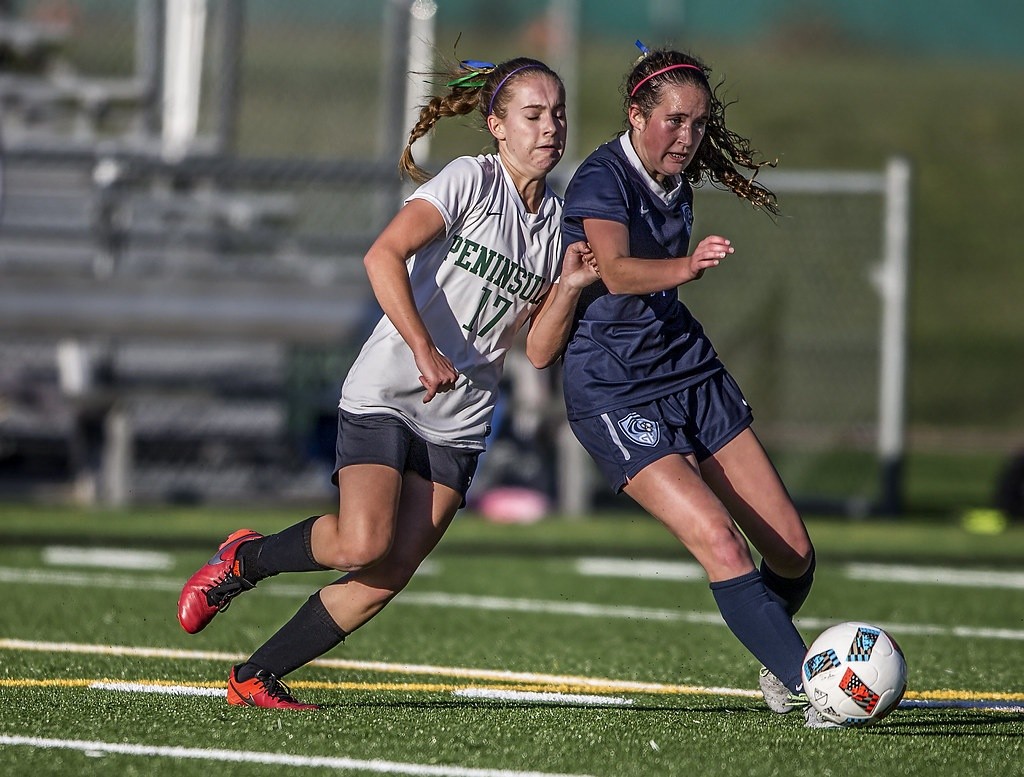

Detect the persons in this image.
[560,36,845,730]
[177,27,604,711]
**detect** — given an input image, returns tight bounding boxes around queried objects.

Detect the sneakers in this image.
[759,663,795,713]
[785,692,847,728]
[175,529,266,635]
[226,663,320,709]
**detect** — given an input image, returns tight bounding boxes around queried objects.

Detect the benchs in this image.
[0,143,553,517]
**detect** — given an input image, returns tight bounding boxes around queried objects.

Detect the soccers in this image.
[801,620,909,730]
